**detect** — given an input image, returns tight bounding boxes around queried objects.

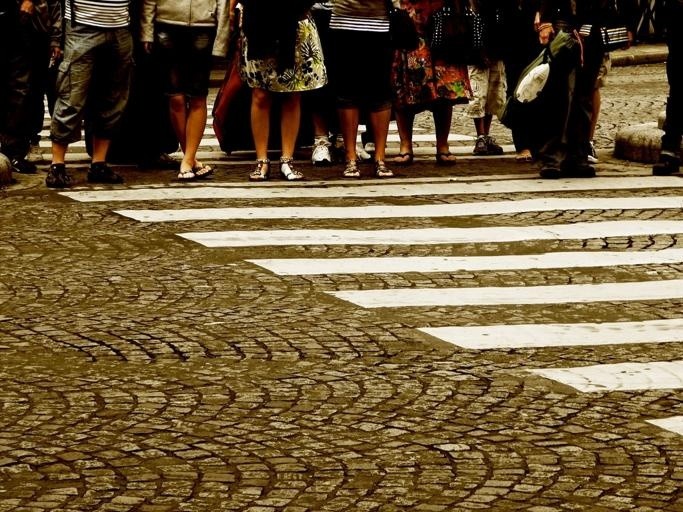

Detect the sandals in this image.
[343,159,361,178]
[249,159,270,180]
[375,152,413,177]
[279,156,304,180]
[473,135,503,154]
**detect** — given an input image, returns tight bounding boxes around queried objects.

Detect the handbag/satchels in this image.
[427,8,486,51]
[389,7,419,50]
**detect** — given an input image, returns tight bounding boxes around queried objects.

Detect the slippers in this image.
[178,162,212,181]
[436,153,456,164]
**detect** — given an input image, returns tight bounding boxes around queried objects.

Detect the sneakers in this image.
[312,136,331,165]
[10,157,37,173]
[87,167,121,183]
[654,154,679,174]
[46,166,70,186]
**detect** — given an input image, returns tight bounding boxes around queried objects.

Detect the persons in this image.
[0,0,682,187]
